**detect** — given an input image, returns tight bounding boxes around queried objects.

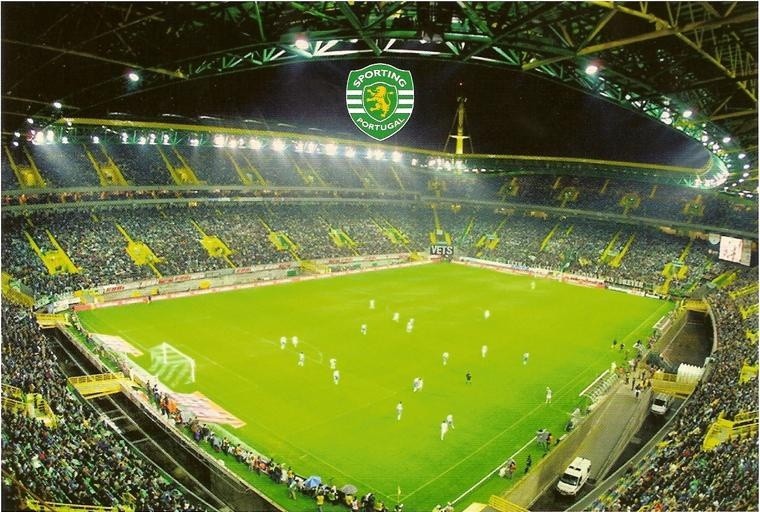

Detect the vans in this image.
[651,392,676,415]
[556,457,592,496]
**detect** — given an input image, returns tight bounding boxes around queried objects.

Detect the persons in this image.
[297,352,305,367]
[413,377,423,392]
[565,419,573,432]
[481,344,488,359]
[329,357,338,371]
[291,335,300,348]
[361,324,368,335]
[332,370,340,385]
[544,387,553,404]
[213,428,580,511]
[580,295,758,512]
[2,296,212,512]
[465,370,473,387]
[572,406,580,426]
[369,299,376,310]
[1,136,758,296]
[442,351,449,368]
[413,376,424,386]
[447,413,455,430]
[392,312,400,324]
[406,318,415,333]
[440,420,449,441]
[483,308,491,321]
[396,401,403,421]
[279,336,288,350]
[522,349,531,366]
